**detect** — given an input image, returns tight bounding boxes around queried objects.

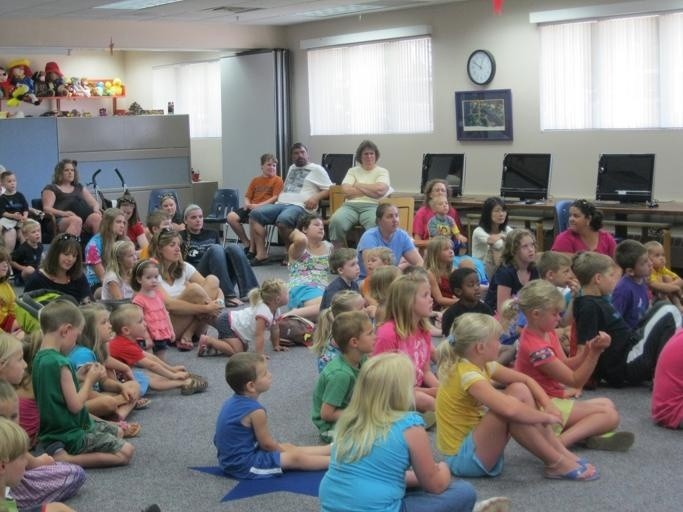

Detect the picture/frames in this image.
[456,89,512,142]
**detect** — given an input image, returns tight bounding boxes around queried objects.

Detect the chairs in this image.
[317,186,357,247]
[236,222,276,257]
[380,198,415,237]
[148,189,182,222]
[29,199,57,240]
[201,189,243,247]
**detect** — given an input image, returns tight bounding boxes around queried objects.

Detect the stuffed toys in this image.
[0,56,122,107]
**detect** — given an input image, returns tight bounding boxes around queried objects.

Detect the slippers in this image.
[177,339,194,351]
[577,456,600,473]
[544,466,601,481]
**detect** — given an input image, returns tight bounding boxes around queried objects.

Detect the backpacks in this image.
[277,313,317,347]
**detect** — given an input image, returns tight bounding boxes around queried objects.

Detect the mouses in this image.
[526,200,535,204]
[649,203,658,208]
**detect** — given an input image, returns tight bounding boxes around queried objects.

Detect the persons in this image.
[1,140,682,511]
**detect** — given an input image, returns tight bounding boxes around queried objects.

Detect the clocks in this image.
[468,49,496,85]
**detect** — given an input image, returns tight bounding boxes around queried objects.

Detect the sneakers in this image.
[186,371,202,379]
[182,377,208,395]
[474,495,513,512]
[587,431,635,451]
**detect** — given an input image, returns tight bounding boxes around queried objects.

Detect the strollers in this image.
[90,166,139,229]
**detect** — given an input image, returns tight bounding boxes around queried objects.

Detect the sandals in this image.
[136,399,151,410]
[113,423,140,438]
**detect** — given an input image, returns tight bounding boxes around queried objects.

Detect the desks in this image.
[392,191,683,270]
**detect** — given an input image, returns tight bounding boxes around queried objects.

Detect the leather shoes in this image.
[244,246,271,266]
[280,254,288,266]
[198,334,207,356]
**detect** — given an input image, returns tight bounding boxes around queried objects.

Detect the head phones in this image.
[423,151,430,170]
[503,152,511,173]
[598,153,607,175]
[323,152,329,168]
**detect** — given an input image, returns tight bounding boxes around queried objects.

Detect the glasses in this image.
[57,234,82,243]
[579,199,587,205]
[162,192,176,198]
[160,226,175,234]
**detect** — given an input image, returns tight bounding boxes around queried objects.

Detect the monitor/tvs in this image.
[421,153,464,194]
[321,154,354,186]
[500,153,551,200]
[596,153,655,204]
